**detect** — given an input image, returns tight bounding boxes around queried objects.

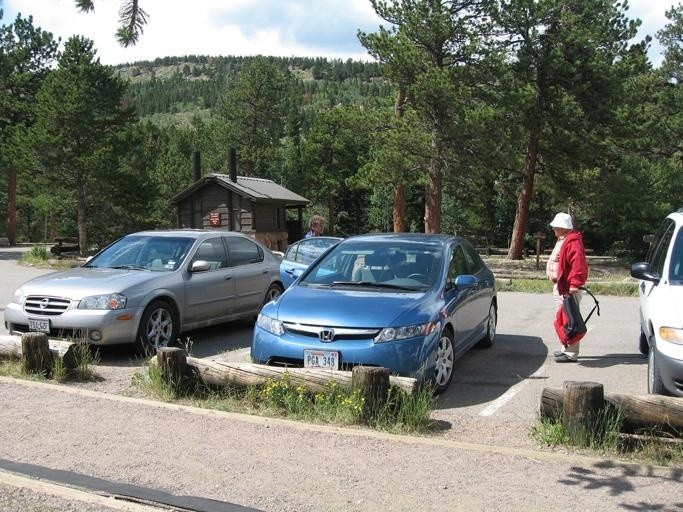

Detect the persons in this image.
[546,212,588,363]
[304,215,332,247]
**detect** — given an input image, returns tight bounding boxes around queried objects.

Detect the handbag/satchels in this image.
[553,295,586,348]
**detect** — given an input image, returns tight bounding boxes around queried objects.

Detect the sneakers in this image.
[553,351,577,362]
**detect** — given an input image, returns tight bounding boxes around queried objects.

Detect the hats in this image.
[549,212,573,230]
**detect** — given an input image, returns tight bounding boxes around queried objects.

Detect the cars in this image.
[250,230,499,398]
[2,226,285,359]
[630,208,683,398]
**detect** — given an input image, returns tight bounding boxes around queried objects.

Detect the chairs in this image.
[358,254,435,284]
